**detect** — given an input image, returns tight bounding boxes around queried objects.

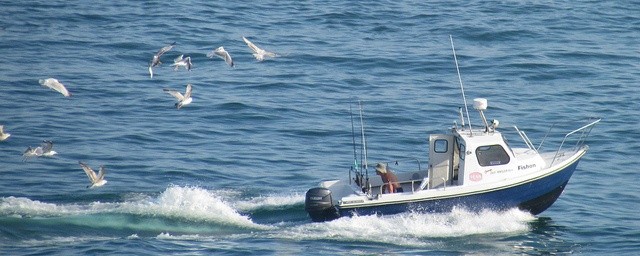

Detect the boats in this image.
[306,34,602,222]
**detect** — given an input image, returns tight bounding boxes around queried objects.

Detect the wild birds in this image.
[39,78,71,97]
[24,140,58,157]
[0,125,10,141]
[169,54,192,71]
[241,36,281,61]
[79,161,108,189]
[207,46,233,67]
[148,42,176,79]
[163,83,193,110]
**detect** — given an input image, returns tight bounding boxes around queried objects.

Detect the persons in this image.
[375,162,404,193]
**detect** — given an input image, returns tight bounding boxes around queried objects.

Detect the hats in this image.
[375,163,387,174]
